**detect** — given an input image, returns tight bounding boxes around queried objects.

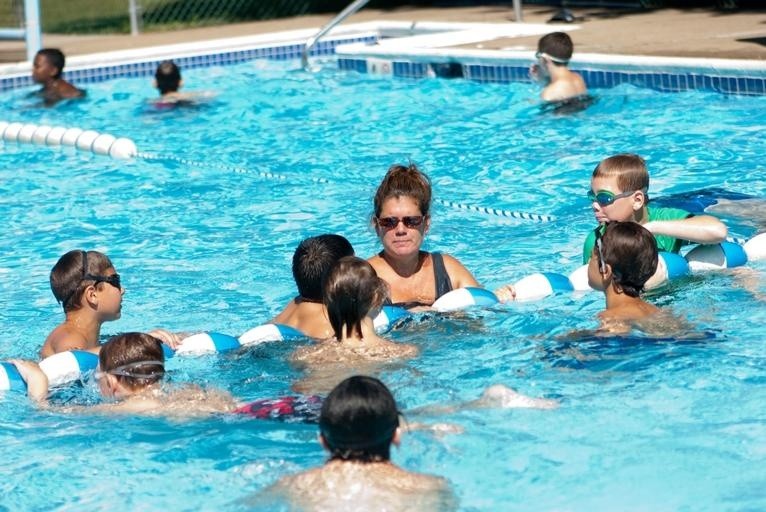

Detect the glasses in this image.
[377,215,425,228]
[80,273,121,291]
[587,186,647,207]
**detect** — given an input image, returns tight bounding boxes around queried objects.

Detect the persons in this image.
[581,220,682,337]
[289,257,420,394]
[261,233,353,340]
[147,63,210,102]
[7,331,562,437]
[225,375,453,511]
[36,250,184,361]
[27,48,84,106]
[363,165,517,314]
[529,32,587,109]
[583,152,728,265]
